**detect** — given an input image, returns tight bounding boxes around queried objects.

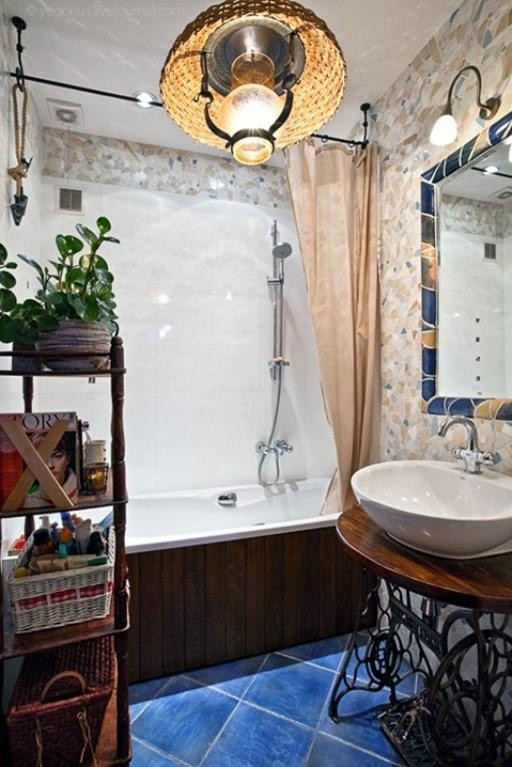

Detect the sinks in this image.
[350,459,512,560]
[37,477,347,557]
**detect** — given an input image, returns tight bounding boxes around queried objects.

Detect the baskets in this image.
[5,526,117,767]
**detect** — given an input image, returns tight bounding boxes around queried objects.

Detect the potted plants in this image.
[1,215,125,373]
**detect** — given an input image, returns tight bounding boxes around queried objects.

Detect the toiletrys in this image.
[34,510,109,557]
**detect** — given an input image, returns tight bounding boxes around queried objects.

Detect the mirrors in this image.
[418,112,510,421]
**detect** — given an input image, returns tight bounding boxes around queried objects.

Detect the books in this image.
[0,412,78,512]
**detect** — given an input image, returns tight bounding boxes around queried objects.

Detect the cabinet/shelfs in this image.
[1,336,130,765]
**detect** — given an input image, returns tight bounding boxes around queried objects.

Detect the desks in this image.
[323,506,512,767]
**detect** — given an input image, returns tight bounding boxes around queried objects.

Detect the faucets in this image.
[435,415,501,472]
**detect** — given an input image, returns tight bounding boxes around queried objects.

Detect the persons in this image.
[23,432,78,508]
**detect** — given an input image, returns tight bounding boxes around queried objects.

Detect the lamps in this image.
[429,61,501,147]
[157,0,349,169]
